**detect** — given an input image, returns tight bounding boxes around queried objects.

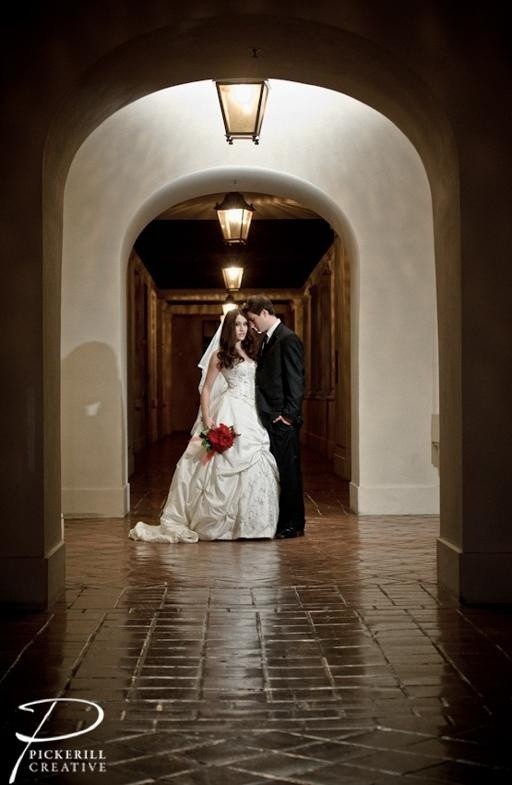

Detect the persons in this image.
[129,303,281,544]
[241,293,307,541]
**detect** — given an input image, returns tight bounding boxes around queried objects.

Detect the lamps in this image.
[213,192,256,247]
[222,258,244,293]
[213,77,271,145]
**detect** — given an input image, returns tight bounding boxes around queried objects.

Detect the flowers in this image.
[201,422,241,463]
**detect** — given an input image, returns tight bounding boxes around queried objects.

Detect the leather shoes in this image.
[274,520,306,539]
